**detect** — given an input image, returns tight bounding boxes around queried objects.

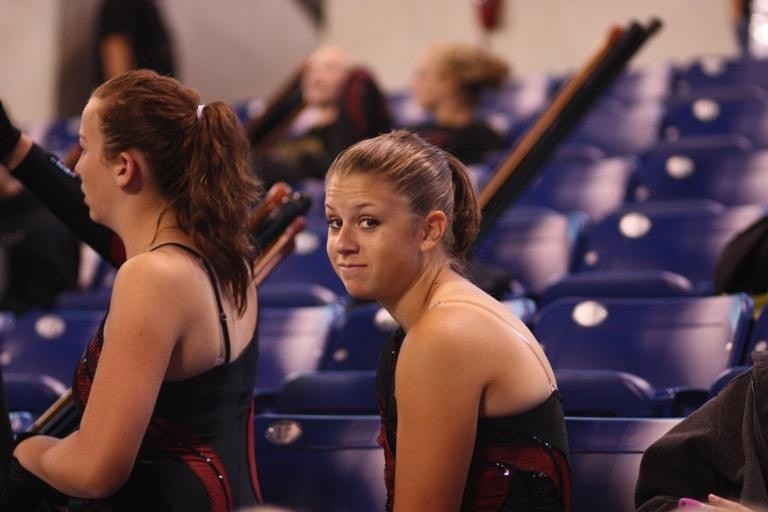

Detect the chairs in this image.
[0,51,768,511]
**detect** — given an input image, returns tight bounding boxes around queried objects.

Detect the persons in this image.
[635,339,765,512]
[402,40,513,165]
[322,129,578,512]
[87,0,182,91]
[0,68,266,510]
[0,164,82,314]
[254,40,386,179]
